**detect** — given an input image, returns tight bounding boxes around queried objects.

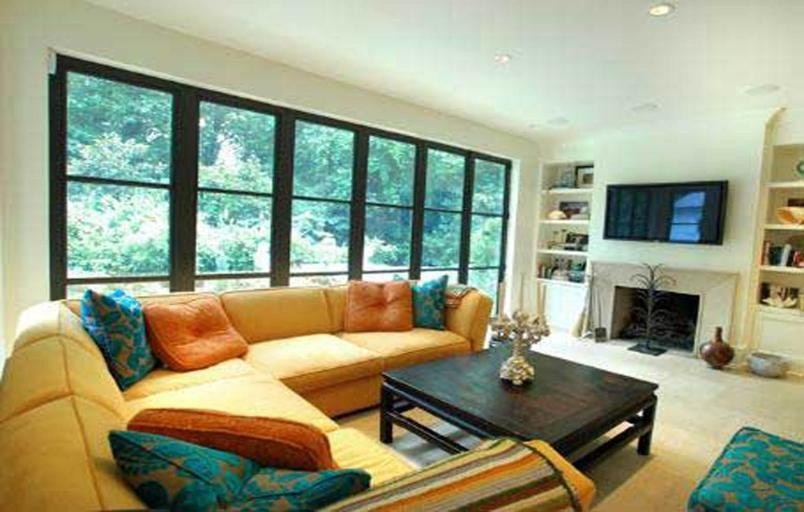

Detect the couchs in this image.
[3,282,597,512]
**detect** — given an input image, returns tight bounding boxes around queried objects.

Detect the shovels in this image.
[595,288,606,343]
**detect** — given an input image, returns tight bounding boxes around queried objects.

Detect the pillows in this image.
[82,285,250,393]
[109,407,374,512]
[348,274,449,332]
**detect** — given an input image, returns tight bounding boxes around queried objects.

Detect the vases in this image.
[701,324,734,370]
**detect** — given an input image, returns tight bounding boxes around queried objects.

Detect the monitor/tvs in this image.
[602,178,729,245]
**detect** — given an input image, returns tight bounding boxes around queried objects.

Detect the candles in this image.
[496,274,549,323]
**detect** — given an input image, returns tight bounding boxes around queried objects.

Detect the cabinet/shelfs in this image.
[750,106,803,373]
[531,148,593,336]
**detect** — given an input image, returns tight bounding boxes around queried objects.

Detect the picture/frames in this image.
[574,165,594,189]
